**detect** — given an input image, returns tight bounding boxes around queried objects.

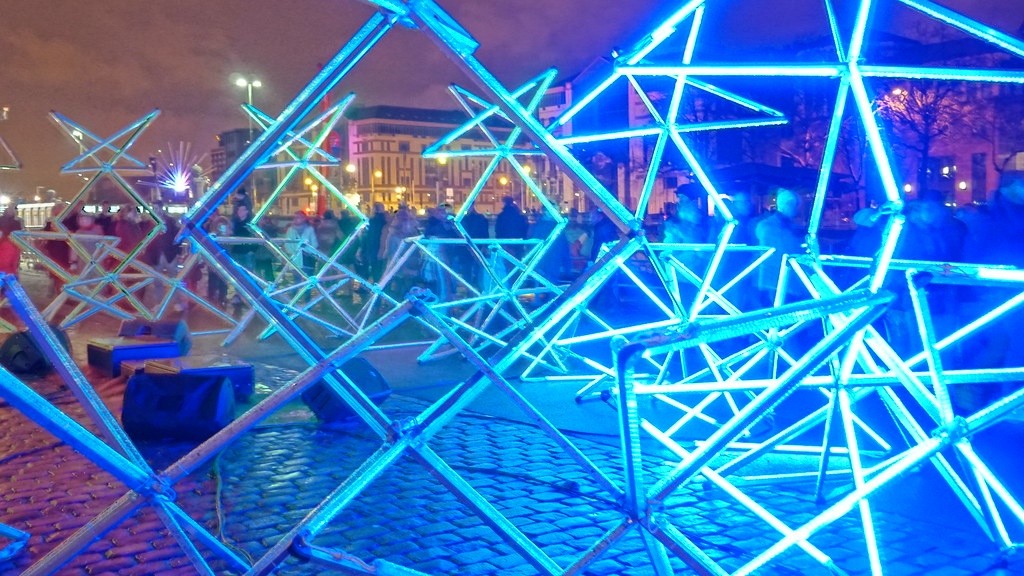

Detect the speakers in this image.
[0,324,69,380]
[299,353,393,421]
[121,371,236,456]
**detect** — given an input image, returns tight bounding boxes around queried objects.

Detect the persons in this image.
[1,165,1023,393]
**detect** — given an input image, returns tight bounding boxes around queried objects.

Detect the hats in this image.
[676,184,695,202]
[295,211,305,217]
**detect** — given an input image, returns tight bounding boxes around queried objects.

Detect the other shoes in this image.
[229,295,241,303]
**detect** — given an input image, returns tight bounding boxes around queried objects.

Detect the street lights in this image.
[371,170,382,205]
[437,157,449,207]
[574,192,582,213]
[501,177,507,198]
[72,131,83,171]
[520,164,530,210]
[237,78,262,214]
[310,184,318,211]
